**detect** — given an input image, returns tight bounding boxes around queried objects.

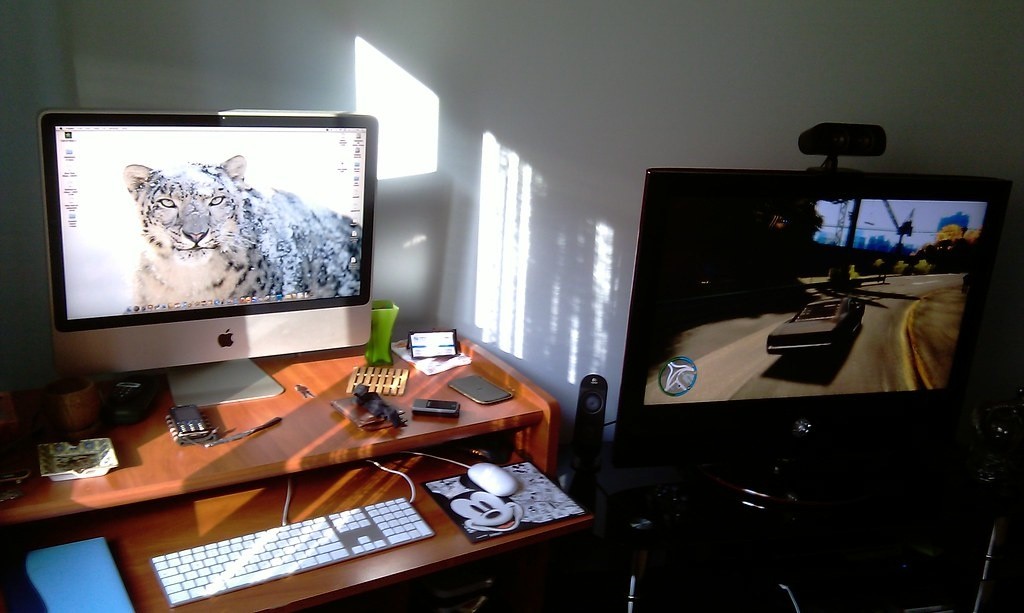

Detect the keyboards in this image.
[151,497,437,608]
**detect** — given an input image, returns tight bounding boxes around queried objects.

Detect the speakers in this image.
[566,374,608,540]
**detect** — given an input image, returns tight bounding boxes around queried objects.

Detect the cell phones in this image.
[412,398,460,418]
[171,404,209,438]
[449,375,512,405]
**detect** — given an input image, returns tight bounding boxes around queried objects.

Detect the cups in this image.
[42,377,101,433]
[364,299,401,367]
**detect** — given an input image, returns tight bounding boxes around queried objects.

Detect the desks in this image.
[0,332,591,613]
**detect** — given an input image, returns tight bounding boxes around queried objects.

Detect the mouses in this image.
[468,463,522,497]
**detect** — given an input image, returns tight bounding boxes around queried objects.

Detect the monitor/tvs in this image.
[39,109,379,408]
[613,166,1014,511]
